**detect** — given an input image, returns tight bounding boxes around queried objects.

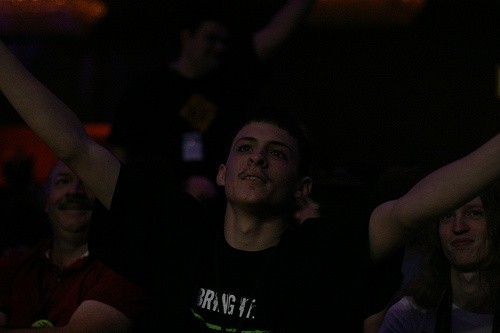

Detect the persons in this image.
[0,39,500,332]
[377,187,500,333]
[113,0,310,191]
[0,158,146,333]
